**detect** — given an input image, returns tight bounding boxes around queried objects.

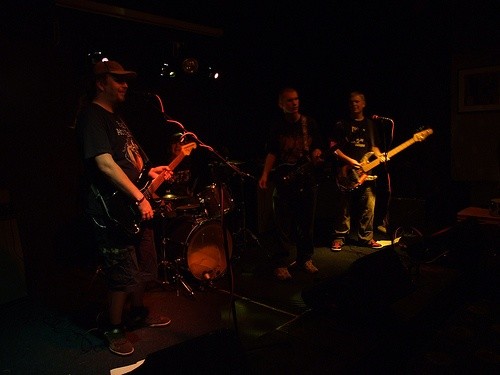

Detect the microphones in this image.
[137,90,157,97]
[369,115,386,119]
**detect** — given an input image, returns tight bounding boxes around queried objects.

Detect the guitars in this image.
[266,126,365,197]
[335,127,433,194]
[89,140,196,238]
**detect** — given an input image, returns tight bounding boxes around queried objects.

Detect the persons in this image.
[255,88,331,281]
[326,91,385,251]
[75,58,173,356]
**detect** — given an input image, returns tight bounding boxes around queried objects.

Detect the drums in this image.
[175,204,202,217]
[157,218,233,282]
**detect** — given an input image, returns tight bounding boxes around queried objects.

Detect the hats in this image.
[95,61,136,75]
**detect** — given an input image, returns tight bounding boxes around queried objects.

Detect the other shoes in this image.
[331,238,344,251]
[296,255,319,274]
[274,266,292,281]
[363,239,381,249]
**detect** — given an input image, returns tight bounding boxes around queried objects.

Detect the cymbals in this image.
[158,194,191,200]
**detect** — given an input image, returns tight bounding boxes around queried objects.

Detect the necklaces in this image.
[135,196,145,206]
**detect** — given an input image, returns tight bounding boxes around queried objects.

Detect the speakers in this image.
[132,327,251,375]
[313,245,410,313]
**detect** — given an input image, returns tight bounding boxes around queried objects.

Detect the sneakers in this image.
[132,307,171,327]
[103,330,135,356]
